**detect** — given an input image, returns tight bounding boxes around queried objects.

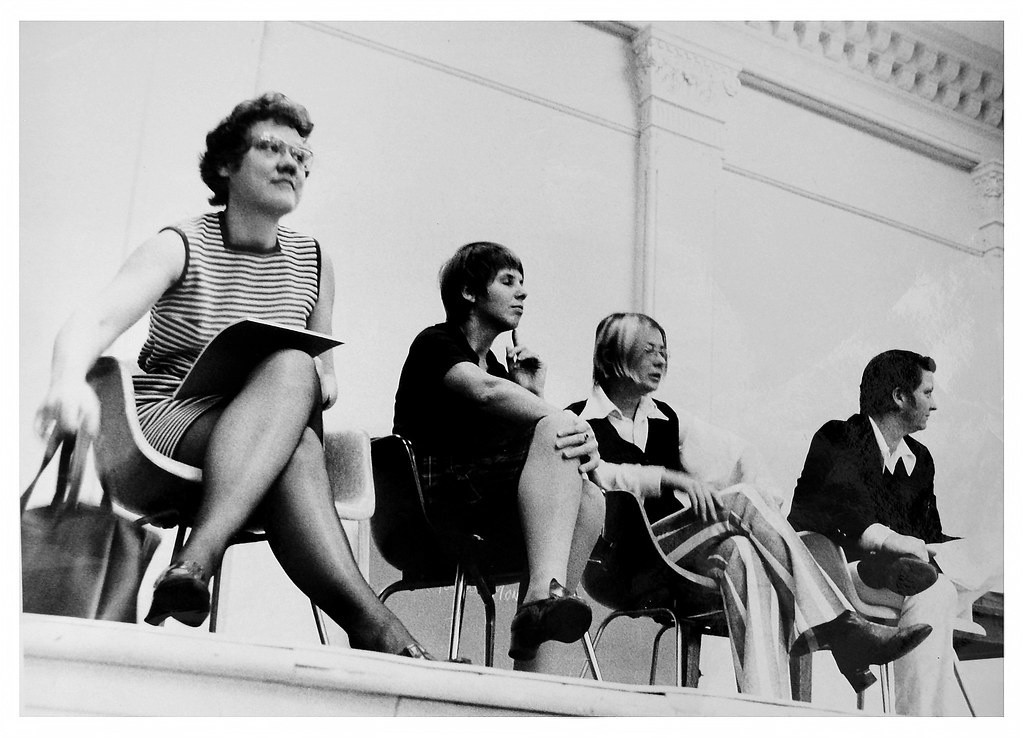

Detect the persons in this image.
[785,349,992,717]
[35,93,432,659]
[393,242,606,675]
[562,312,933,702]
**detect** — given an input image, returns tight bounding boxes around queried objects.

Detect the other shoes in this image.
[856,551,938,596]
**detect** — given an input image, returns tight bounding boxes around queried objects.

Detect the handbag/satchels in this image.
[20,422,177,624]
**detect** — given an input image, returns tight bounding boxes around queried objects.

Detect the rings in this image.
[583,433,589,442]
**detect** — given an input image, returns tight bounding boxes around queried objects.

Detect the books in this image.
[171,317,346,401]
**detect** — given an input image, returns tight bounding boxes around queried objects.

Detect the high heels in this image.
[823,611,932,694]
[144,560,211,628]
[508,597,592,661]
[400,645,472,665]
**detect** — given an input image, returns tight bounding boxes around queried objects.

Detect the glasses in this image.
[642,342,668,362]
[250,134,314,172]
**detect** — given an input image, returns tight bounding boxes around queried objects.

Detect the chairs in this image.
[588,480,726,686]
[365,433,604,681]
[790,530,979,717]
[90,355,378,646]
